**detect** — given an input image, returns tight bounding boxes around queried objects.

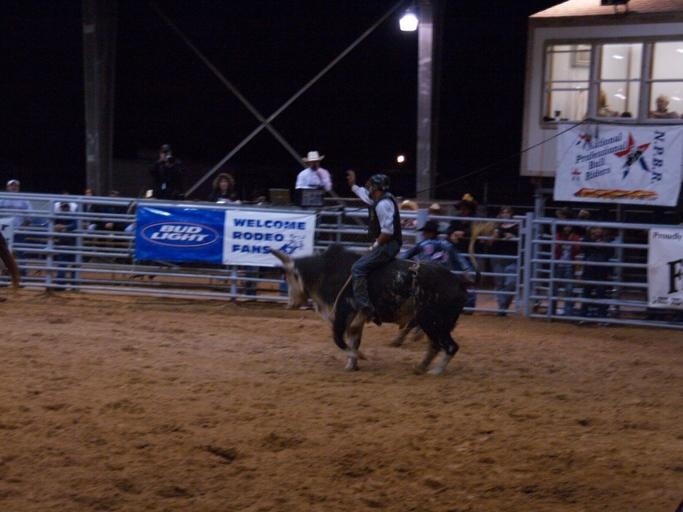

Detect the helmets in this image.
[365,174,390,194]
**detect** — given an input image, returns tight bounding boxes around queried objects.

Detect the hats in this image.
[427,203,442,213]
[302,150,325,163]
[416,221,439,234]
[212,172,232,189]
[158,144,171,152]
[400,199,417,210]
[454,193,477,210]
[6,178,20,186]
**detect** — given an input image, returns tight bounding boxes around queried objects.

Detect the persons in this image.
[584,89,619,117]
[0,148,299,302]
[293,150,618,331]
[650,94,678,119]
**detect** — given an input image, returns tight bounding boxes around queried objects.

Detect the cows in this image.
[270,220,498,379]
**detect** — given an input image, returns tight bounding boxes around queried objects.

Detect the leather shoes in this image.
[351,304,375,328]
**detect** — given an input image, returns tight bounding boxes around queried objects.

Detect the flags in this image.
[552,124,681,209]
